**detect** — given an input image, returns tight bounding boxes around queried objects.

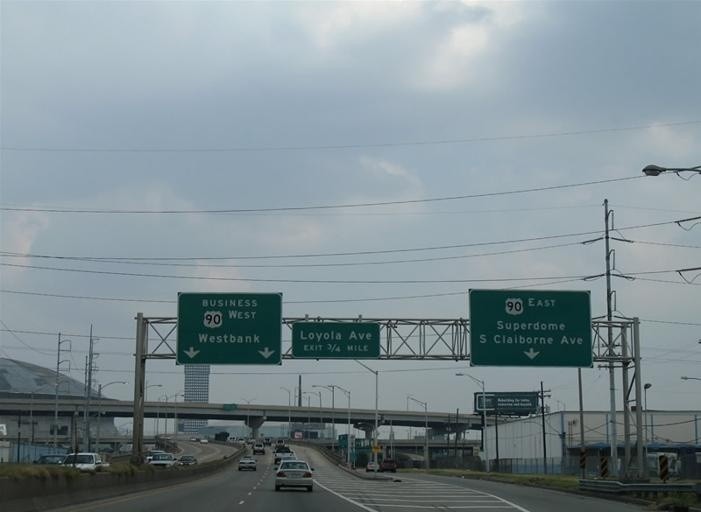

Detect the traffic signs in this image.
[290,321,380,360]
[466,288,595,369]
[174,291,283,366]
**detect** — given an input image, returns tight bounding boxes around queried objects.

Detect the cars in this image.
[189,436,297,471]
[365,460,380,472]
[274,459,314,493]
[32,449,198,472]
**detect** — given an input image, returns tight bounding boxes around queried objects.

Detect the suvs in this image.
[379,458,397,472]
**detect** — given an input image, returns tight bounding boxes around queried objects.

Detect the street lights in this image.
[410,397,429,469]
[640,162,700,179]
[280,382,379,466]
[643,382,653,461]
[406,392,415,411]
[680,375,700,382]
[236,397,256,440]
[454,373,489,472]
[30,379,186,448]
